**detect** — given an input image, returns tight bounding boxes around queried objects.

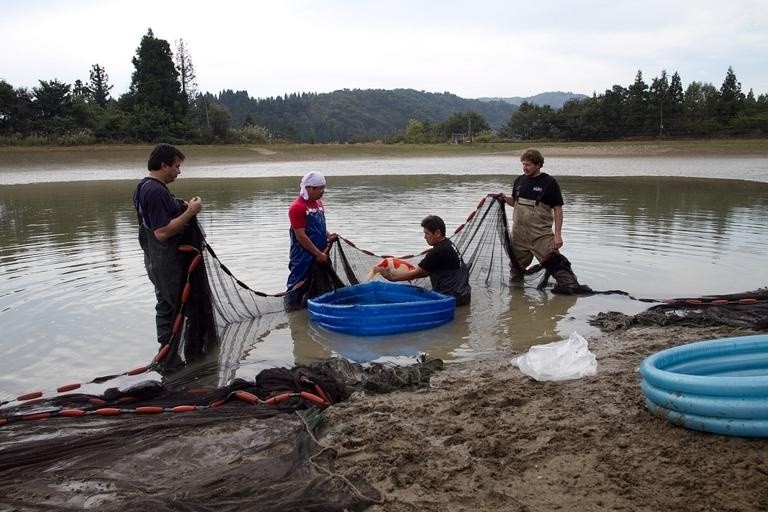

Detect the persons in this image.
[490,149,579,295]
[379,211,471,305]
[133,142,216,368]
[283,172,337,314]
